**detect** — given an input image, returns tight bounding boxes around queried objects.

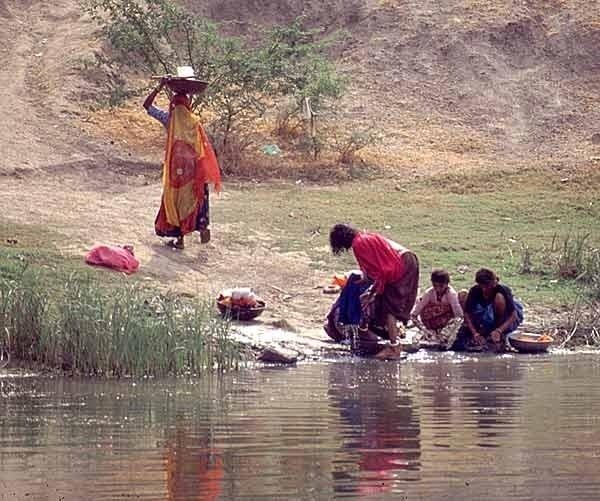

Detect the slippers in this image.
[166,239,185,250]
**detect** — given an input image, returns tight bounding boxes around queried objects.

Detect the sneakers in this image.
[200,229,211,243]
[374,342,403,361]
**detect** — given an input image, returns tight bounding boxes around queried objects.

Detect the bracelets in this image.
[154,86,161,93]
[495,328,504,335]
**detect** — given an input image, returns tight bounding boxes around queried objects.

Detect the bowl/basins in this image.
[166,78,208,95]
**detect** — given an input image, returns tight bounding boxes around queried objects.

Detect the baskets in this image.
[151,75,210,94]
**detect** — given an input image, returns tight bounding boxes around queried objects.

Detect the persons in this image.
[144,76,222,250]
[329,222,421,360]
[410,270,464,350]
[463,266,524,353]
